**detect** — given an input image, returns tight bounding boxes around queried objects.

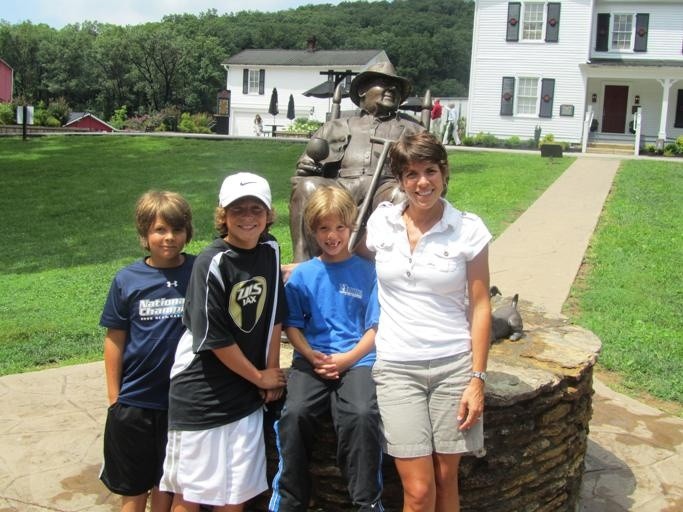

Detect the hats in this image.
[218,172,272,213]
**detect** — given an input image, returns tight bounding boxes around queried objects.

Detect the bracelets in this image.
[469,371,486,383]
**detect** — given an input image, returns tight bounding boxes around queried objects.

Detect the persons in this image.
[156,170,288,512]
[101,187,199,512]
[344,129,491,511]
[430,98,443,143]
[288,61,429,260]
[442,103,462,145]
[279,182,386,510]
